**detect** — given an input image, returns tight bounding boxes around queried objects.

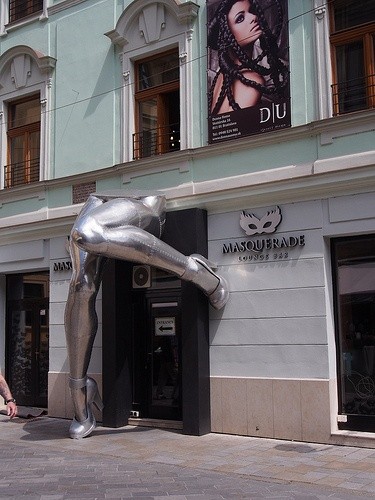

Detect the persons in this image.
[207,0,290,117]
[0,374,18,419]
[64,196,229,440]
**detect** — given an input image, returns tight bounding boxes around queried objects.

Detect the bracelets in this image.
[5,399,16,405]
